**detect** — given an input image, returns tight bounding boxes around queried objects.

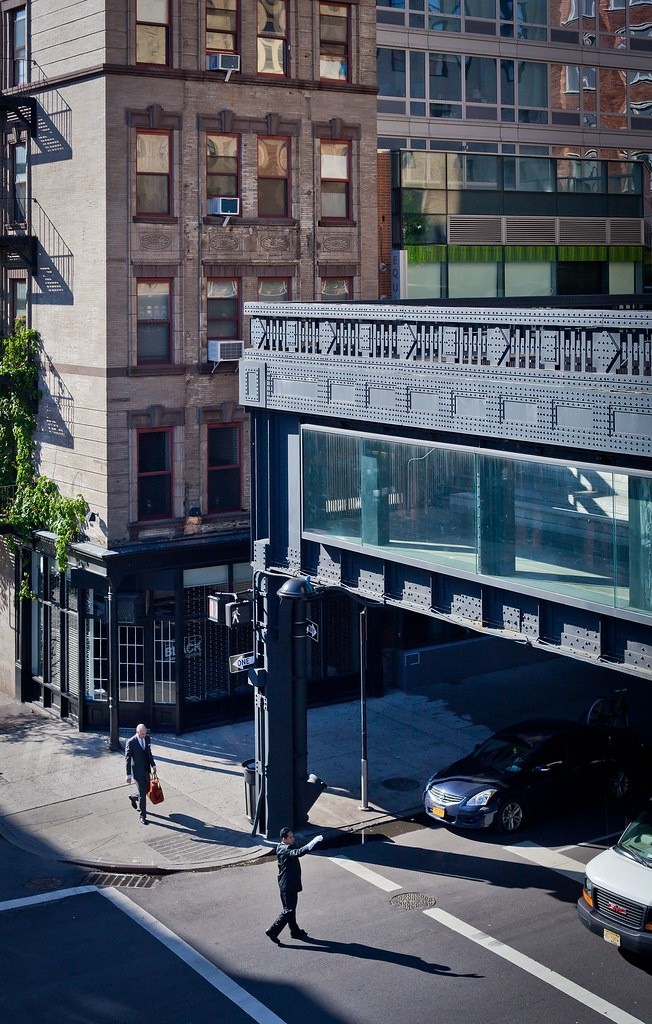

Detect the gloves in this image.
[306,835,323,851]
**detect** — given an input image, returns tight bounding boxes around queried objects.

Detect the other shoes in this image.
[140,818,148,825]
[290,930,308,938]
[129,795,137,809]
[266,930,280,943]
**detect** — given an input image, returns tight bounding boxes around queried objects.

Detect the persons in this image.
[491,743,533,772]
[124,723,158,825]
[265,827,324,945]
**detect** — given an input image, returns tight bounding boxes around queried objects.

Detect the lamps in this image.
[88,512,99,522]
[276,579,321,602]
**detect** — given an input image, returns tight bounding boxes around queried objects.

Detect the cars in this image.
[421,719,642,837]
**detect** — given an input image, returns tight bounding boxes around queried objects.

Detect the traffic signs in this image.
[229,651,258,673]
[306,618,319,642]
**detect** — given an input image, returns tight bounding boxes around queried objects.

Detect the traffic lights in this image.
[226,601,252,630]
[208,597,224,623]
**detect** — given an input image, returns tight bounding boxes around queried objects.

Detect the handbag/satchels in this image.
[146,773,164,805]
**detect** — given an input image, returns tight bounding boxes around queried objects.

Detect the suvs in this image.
[578,798,652,957]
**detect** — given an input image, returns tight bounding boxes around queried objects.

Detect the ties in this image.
[141,739,144,748]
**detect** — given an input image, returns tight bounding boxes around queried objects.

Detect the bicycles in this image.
[586,687,633,729]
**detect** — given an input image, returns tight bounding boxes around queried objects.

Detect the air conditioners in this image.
[212,197,239,215]
[209,54,239,71]
[208,340,244,362]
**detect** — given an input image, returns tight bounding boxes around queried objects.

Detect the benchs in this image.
[449,492,629,546]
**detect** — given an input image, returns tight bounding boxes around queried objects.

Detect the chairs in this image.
[640,834,652,845]
